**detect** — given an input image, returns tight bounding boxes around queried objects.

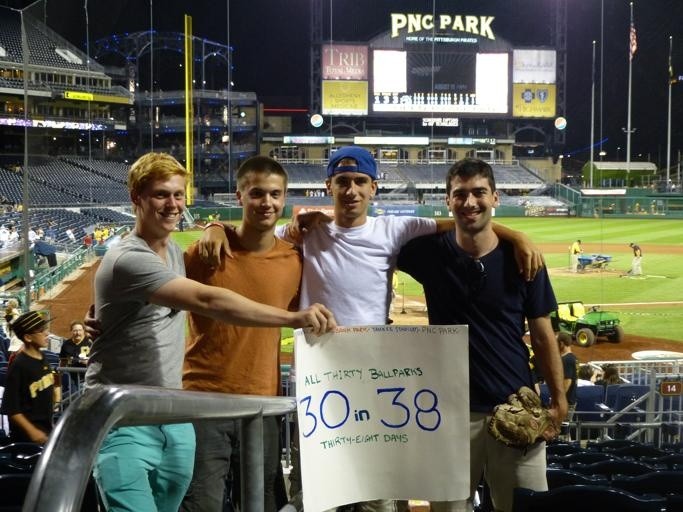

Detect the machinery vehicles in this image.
[576,254,611,271]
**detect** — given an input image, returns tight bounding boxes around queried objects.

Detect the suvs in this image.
[548,301,624,346]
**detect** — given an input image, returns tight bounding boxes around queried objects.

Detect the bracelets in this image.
[202,223,225,229]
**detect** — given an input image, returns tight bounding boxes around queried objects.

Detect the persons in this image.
[84,150,337,512]
[303,187,325,199]
[55,321,89,411]
[0,222,43,254]
[178,209,221,232]
[628,242,644,275]
[47,217,56,228]
[523,330,624,445]
[0,311,59,448]
[196,150,545,512]
[0,299,25,368]
[82,226,115,249]
[0,202,22,216]
[80,154,302,511]
[567,240,584,275]
[283,160,571,512]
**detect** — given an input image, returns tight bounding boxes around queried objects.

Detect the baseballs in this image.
[311,114,323,127]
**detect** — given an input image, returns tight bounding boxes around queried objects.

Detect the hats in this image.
[326,146,376,179]
[12,311,56,336]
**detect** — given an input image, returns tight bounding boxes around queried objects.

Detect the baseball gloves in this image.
[488,387,560,451]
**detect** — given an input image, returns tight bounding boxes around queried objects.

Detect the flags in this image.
[666,58,676,86]
[626,21,637,63]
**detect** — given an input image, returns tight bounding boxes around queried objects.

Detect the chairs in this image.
[279,157,543,203]
[0,327,110,512]
[475,378,683,512]
[0,3,136,248]
